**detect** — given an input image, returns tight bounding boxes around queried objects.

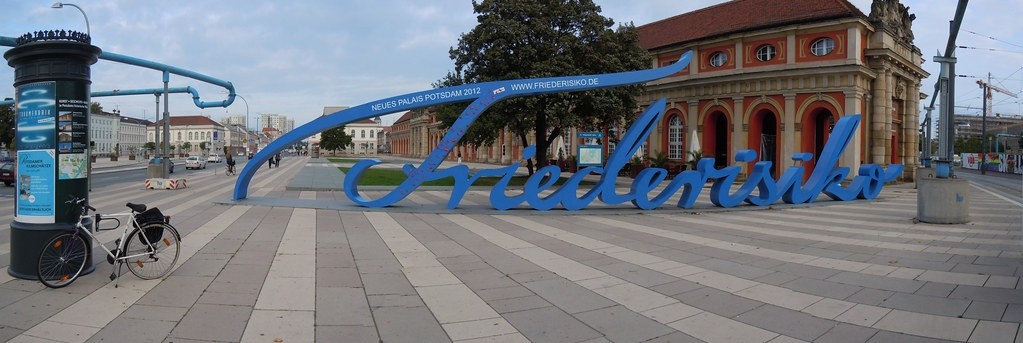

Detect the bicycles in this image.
[225,161,237,176]
[37,196,181,289]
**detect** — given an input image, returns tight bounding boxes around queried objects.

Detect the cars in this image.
[185,156,206,170]
[208,153,221,163]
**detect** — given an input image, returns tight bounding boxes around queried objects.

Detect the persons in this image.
[458,151,461,163]
[226,151,233,172]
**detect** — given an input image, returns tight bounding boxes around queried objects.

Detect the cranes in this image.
[976,72,1021,117]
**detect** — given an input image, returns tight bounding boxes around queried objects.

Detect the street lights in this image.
[229,94,249,162]
[51,1,92,191]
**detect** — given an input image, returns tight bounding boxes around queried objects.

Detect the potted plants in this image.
[648,149,676,168]
[683,149,714,170]
[631,155,645,178]
[568,154,577,173]
[556,147,566,172]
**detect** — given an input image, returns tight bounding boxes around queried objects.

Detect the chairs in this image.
[651,164,687,180]
[619,163,630,177]
